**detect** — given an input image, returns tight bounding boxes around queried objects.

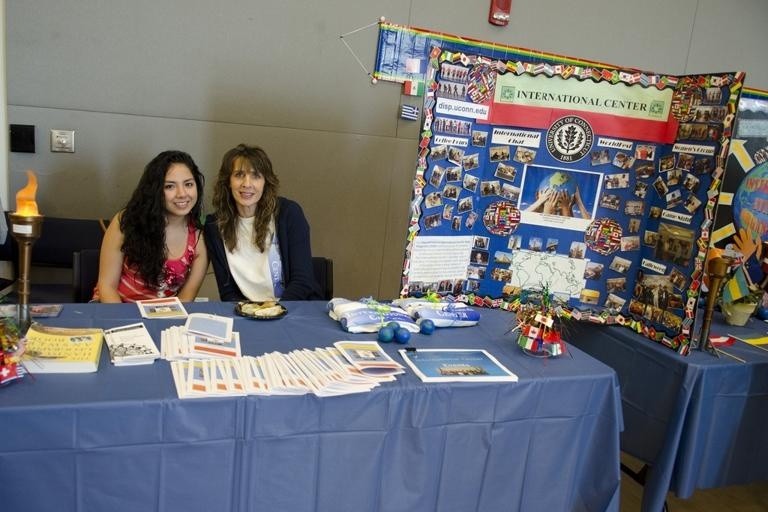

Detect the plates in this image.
[234,301,288,320]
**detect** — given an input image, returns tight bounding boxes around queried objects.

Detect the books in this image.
[15,321,104,374]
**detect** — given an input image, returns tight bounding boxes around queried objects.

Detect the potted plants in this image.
[723,287,765,327]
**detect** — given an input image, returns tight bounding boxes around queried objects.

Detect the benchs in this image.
[0,210,111,303]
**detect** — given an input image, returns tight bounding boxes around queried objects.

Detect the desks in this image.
[2,298,631,512]
[553,299,766,511]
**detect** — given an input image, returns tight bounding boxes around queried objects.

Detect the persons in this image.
[430,145,590,230]
[202,143,315,300]
[91,149,208,305]
[435,117,473,137]
[438,64,467,97]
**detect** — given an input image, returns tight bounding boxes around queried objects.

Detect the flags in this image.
[445,51,690,90]
[723,266,750,304]
[743,253,766,287]
[692,75,749,335]
[706,333,736,348]
[422,290,690,360]
[732,332,768,346]
[394,46,442,299]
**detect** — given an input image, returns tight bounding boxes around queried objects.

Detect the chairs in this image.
[79,247,124,303]
[311,257,334,301]
